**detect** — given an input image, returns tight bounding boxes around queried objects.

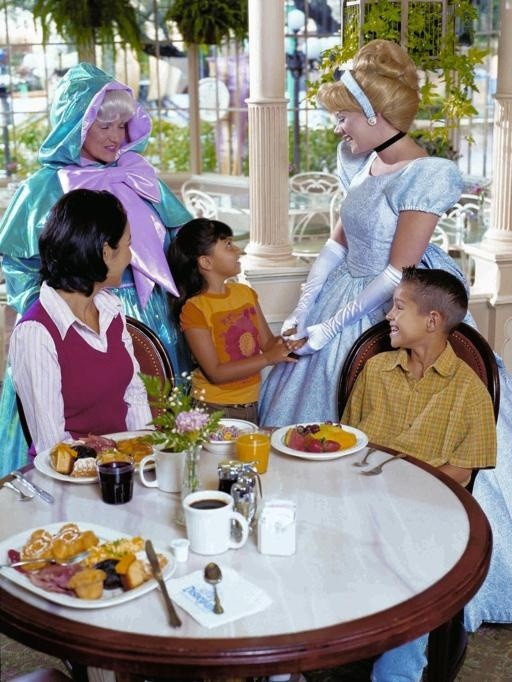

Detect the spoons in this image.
[203,559,226,614]
[3,478,35,503]
[1,548,92,570]
[353,447,377,468]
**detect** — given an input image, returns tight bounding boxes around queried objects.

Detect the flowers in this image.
[136,370,226,497]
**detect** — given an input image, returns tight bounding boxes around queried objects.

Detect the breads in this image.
[118,437,154,461]
[51,444,75,474]
[19,522,147,601]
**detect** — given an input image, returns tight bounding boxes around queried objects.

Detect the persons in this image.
[0,61,193,480]
[8,188,156,464]
[168,218,307,426]
[339,264,497,682]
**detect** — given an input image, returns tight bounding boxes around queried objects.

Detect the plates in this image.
[0,520,176,610]
[33,430,153,483]
[270,422,366,461]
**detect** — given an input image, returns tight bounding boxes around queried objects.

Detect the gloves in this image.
[280,238,348,341]
[289,264,403,357]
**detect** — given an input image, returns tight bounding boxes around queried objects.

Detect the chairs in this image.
[288,172,342,245]
[4,667,80,681]
[181,179,220,225]
[338,320,500,682]
[16,315,175,449]
[427,172,491,261]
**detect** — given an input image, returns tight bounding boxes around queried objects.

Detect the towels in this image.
[157,567,273,628]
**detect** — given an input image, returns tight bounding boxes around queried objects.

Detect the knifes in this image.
[10,469,58,505]
[144,539,182,629]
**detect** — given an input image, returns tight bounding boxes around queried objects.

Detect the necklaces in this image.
[257,39,512,635]
[373,131,407,153]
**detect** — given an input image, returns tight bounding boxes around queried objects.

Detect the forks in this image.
[361,452,407,478]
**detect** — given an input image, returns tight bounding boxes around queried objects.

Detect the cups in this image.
[217,459,259,537]
[142,441,189,494]
[183,490,249,556]
[258,498,297,556]
[169,539,190,563]
[95,452,138,506]
[234,431,271,474]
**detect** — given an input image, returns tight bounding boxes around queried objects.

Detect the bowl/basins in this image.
[200,417,260,454]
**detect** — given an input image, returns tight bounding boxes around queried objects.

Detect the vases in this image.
[175,449,203,527]
